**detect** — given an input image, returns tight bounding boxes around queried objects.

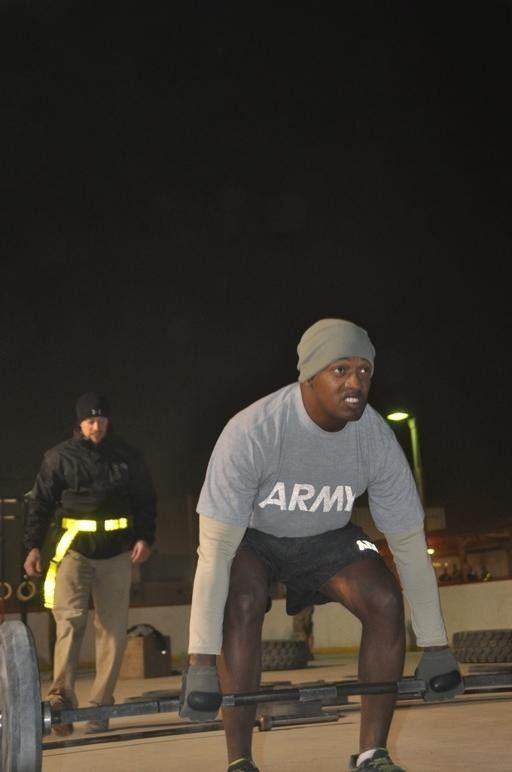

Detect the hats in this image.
[76,393,110,423]
[296,318,374,382]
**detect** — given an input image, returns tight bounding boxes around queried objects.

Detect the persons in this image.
[437,559,489,585]
[177,317,468,770]
[20,390,159,740]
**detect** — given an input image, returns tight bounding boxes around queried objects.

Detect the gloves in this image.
[413,651,462,703]
[178,666,221,722]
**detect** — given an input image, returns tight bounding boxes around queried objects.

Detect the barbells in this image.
[0,615,512,772]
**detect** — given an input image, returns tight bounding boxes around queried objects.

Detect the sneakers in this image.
[348,748,407,772]
[84,717,105,734]
[48,694,72,738]
